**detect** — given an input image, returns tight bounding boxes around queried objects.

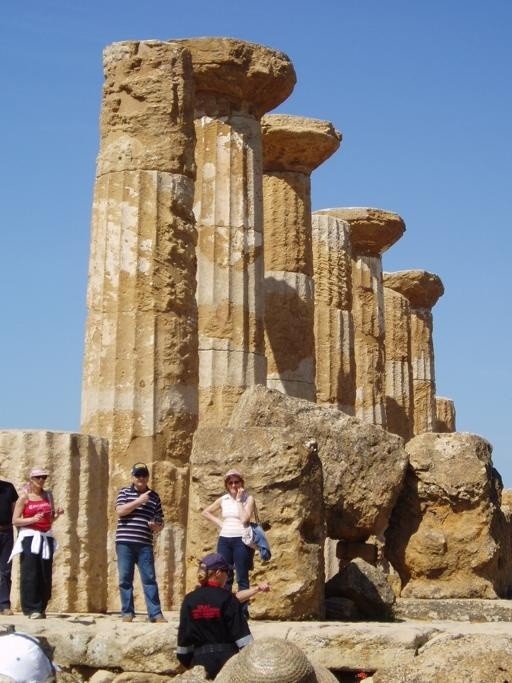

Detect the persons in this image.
[12,467,64,619]
[37,636,62,671]
[234,582,271,604]
[201,469,255,621]
[116,462,168,623]
[177,553,254,678]
[0,463,22,616]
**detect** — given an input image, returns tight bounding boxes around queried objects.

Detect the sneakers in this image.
[2,608,13,615]
[152,618,168,623]
[29,611,47,619]
[121,615,132,622]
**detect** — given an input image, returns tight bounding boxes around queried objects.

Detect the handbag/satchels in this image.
[240,525,258,550]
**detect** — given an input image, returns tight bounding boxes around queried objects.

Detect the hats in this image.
[199,552,238,571]
[223,469,242,482]
[130,462,149,474]
[30,467,50,478]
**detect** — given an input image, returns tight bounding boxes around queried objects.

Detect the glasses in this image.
[228,480,240,484]
[134,473,147,478]
[35,475,46,479]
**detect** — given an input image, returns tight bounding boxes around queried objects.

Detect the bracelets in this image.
[237,497,242,502]
